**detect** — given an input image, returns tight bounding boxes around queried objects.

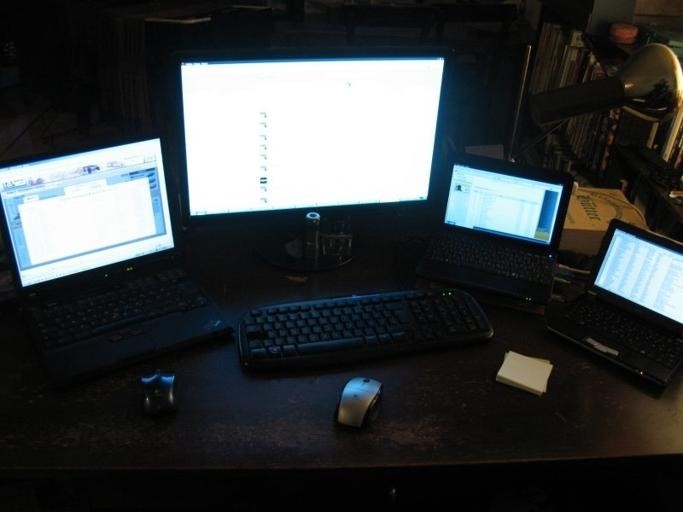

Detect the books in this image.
[530,21,683,178]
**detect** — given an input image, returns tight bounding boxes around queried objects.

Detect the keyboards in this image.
[236,288,495,372]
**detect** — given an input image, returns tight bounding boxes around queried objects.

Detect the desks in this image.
[1,237,683,512]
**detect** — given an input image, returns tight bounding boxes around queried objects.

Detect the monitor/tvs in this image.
[175,47,453,272]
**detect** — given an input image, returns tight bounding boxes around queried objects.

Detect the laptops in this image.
[544,218,683,389]
[416,149,574,307]
[0,134,235,391]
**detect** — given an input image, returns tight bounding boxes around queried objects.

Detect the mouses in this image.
[333,376,384,433]
[135,368,180,426]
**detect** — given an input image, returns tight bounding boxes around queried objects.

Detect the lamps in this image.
[503,41,683,172]
